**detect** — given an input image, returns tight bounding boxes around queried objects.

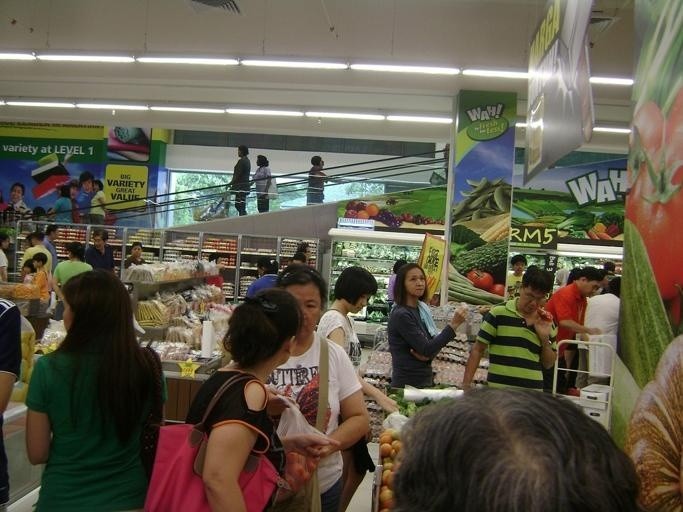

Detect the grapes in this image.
[369,209,401,227]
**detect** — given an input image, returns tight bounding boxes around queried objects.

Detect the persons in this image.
[0,171,94,223]
[91,180,108,223]
[307,156,330,205]
[225,145,251,215]
[250,155,273,213]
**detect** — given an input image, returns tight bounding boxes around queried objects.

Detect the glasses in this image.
[523,291,549,304]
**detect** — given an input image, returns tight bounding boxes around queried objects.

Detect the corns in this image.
[480,216,509,243]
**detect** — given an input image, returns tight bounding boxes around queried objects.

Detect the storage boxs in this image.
[579,383,611,410]
[582,405,609,432]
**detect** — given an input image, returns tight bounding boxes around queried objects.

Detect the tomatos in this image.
[624,88,683,327]
[466,269,504,296]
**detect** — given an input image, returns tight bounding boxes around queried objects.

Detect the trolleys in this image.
[551,334,613,433]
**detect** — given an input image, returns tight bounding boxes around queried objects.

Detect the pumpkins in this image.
[624,333,683,512]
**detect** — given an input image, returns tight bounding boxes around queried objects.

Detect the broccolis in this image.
[451,225,484,259]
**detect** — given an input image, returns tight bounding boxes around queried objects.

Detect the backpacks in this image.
[264,177,280,200]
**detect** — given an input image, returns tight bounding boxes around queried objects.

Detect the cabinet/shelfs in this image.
[552,241,624,294]
[13,219,320,305]
[510,247,552,271]
[327,228,425,343]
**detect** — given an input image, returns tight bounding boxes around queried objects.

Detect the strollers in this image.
[192,185,232,222]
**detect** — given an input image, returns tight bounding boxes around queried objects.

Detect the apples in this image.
[344,210,357,218]
[346,201,358,210]
[355,202,367,210]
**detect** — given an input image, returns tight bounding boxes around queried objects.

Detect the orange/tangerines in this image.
[379,429,402,512]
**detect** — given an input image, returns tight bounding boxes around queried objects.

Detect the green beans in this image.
[452,177,512,221]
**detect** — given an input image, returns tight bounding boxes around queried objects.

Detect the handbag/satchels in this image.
[143,372,281,512]
[268,469,322,512]
